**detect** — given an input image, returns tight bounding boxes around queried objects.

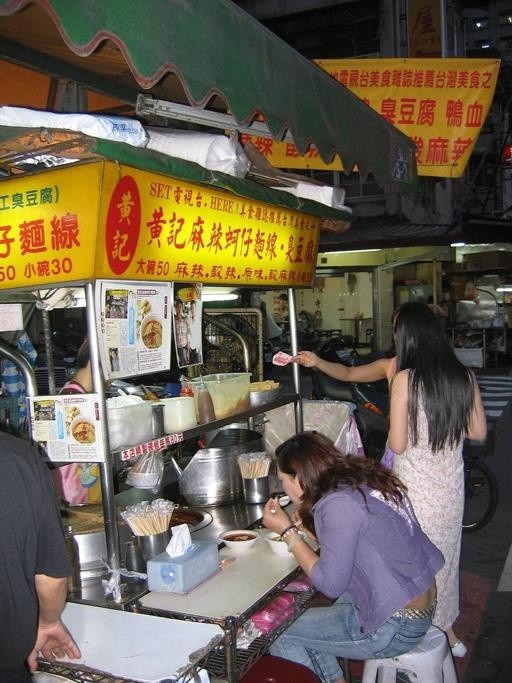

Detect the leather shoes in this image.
[448,640,467,659]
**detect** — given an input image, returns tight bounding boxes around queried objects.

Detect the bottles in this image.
[123,539,147,580]
[180,380,195,397]
[197,374,217,425]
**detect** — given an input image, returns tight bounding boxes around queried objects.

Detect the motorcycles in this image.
[312,333,395,457]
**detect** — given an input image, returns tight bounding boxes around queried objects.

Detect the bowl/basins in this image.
[216,529,260,550]
[128,471,162,491]
[263,530,308,558]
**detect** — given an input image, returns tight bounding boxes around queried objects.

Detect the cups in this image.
[240,474,270,505]
[129,529,171,564]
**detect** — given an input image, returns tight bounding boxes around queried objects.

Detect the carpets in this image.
[338,569,492,682]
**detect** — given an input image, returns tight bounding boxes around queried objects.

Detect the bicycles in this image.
[461,386,499,531]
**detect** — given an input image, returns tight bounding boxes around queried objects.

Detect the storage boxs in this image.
[189,372,252,424]
[106,401,154,452]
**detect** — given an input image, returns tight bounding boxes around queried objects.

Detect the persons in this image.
[388,300,488,658]
[425,292,488,330]
[287,302,403,472]
[186,298,205,364]
[46,333,115,507]
[173,297,194,366]
[297,310,313,334]
[1,432,84,683]
[260,428,447,683]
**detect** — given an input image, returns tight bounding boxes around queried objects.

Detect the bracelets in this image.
[286,533,306,554]
[278,524,298,542]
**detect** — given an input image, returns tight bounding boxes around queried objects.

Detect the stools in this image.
[362,625,459,683]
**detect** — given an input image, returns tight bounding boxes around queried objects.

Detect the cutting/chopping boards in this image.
[54,501,135,565]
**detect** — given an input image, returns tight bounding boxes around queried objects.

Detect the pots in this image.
[170,427,268,509]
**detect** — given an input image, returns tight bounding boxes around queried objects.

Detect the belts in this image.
[393,607,434,618]
